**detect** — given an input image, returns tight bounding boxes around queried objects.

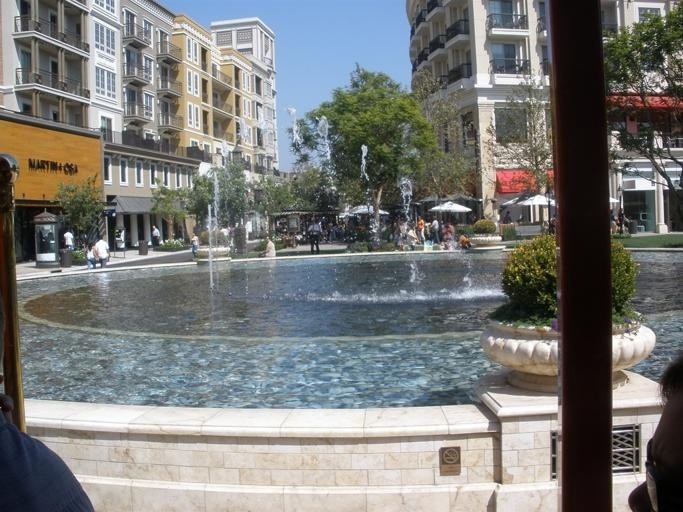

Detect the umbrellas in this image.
[427,200,472,217]
[502,192,555,222]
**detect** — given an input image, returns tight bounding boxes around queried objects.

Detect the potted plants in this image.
[479,231,656,393]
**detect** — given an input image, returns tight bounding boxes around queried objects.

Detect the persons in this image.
[503,211,513,224]
[610,209,618,234]
[429,216,440,243]
[442,222,453,241]
[63,228,76,252]
[92,234,109,268]
[627,355,683,511]
[190,230,198,255]
[618,207,629,234]
[458,232,471,248]
[85,243,96,269]
[307,219,320,253]
[151,225,160,248]
[416,215,425,242]
[258,236,276,257]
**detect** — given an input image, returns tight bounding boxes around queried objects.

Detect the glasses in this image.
[645,439,683,511]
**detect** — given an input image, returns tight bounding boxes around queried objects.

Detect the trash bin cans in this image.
[59,248,72,268]
[138,240,149,255]
